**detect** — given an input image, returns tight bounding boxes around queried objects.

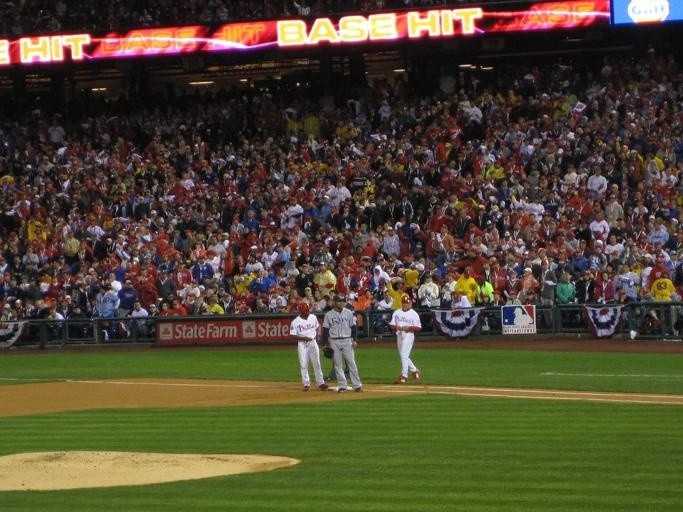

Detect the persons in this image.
[288,303,328,392]
[1,1,681,343]
[322,294,362,393]
[317,307,359,382]
[388,295,422,383]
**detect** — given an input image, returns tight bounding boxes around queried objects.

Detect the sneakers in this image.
[304,374,361,392]
[395,375,407,384]
[414,368,420,380]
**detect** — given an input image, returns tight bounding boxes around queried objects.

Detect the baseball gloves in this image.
[323,347,334,359]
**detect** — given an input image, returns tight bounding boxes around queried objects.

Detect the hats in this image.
[0,46,683,318]
